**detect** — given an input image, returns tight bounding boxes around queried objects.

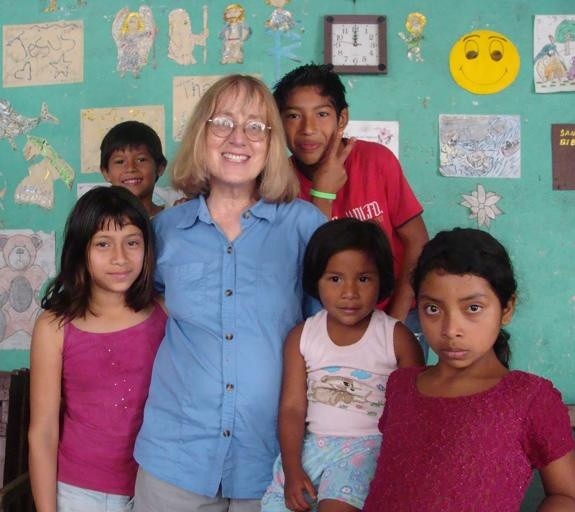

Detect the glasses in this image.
[207,116,272,142]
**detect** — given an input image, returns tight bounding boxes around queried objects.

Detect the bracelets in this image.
[310,189,336,200]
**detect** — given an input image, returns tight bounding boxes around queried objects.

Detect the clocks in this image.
[322,13,387,76]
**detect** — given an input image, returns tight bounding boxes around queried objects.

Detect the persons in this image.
[125,74,331,511]
[218,4,250,65]
[99,120,190,219]
[273,64,429,365]
[260,217,425,512]
[363,226,574,511]
[27,185,172,511]
